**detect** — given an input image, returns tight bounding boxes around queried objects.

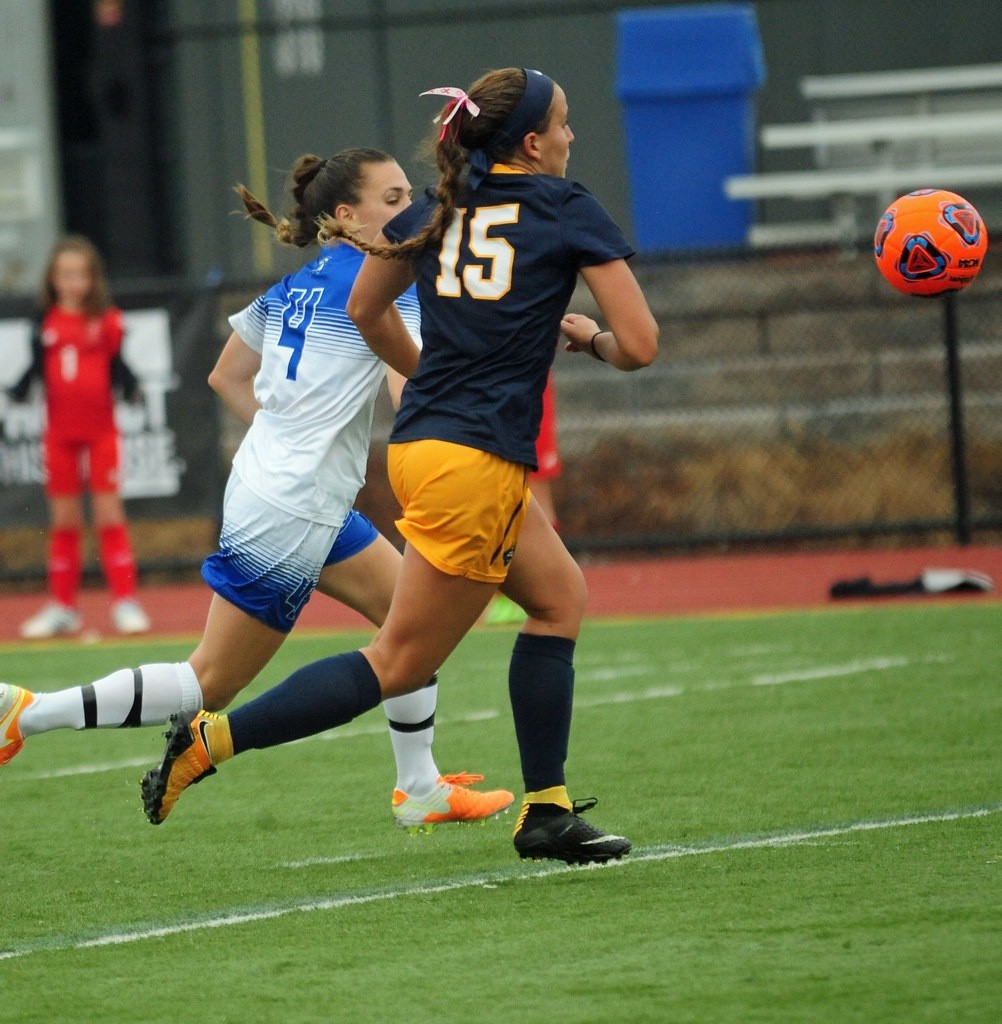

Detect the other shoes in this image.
[21,603,80,638]
[110,601,150,633]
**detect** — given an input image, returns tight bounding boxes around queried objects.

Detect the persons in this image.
[0,147,516,828]
[139,67,659,868]
[4,237,152,640]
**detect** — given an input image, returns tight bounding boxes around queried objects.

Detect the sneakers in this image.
[139,709,217,824]
[391,770,514,836]
[512,798,633,866]
[0,684,36,767]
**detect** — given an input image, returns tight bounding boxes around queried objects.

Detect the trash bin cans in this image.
[613,2,769,253]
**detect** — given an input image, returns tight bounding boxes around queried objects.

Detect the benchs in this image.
[724,63,1002,252]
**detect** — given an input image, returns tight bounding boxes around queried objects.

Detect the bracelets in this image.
[591,331,608,363]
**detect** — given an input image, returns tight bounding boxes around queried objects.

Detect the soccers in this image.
[874,187,987,302]
